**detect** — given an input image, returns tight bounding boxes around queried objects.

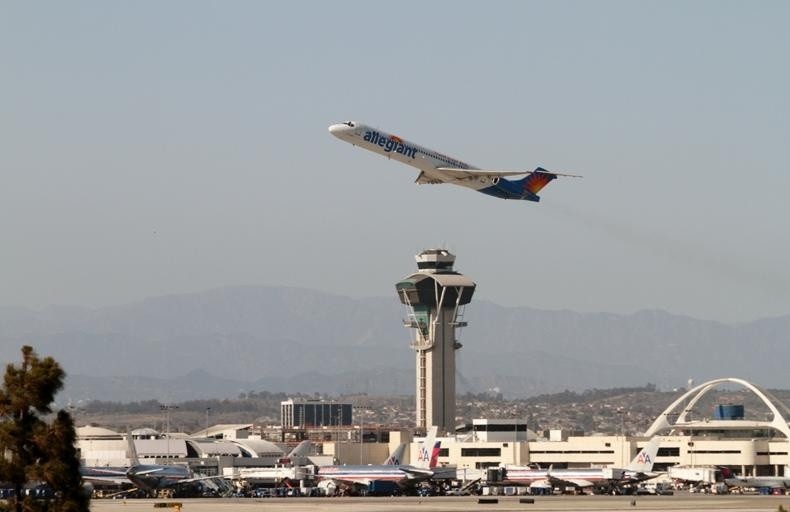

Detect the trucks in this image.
[669,465,723,484]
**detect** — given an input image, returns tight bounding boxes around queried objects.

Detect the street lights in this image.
[353,406,373,464]
[159,405,179,467]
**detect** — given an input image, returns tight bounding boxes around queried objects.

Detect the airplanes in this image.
[328,120,584,202]
